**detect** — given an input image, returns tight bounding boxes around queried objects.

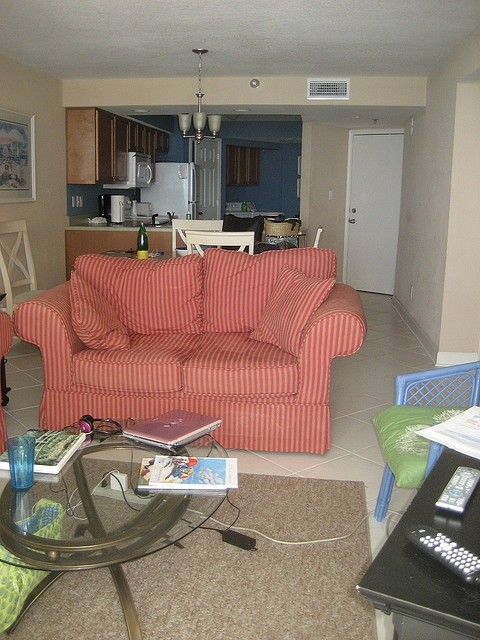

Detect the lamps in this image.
[178,48,222,142]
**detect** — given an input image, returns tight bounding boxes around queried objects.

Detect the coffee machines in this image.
[98,194,111,222]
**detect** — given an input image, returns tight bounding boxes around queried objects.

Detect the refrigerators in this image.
[140,162,197,220]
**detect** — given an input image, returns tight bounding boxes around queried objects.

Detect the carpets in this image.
[0,457,378,640]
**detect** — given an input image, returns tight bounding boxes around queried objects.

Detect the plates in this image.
[174,247,199,257]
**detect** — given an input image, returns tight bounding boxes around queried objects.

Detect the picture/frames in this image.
[1,105,37,203]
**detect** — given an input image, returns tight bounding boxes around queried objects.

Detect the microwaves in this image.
[103,150,153,190]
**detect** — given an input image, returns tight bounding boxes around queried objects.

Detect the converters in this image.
[220,527,257,551]
[110,471,128,492]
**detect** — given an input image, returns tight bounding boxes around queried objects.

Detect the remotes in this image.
[435,465,480,513]
[405,523,480,586]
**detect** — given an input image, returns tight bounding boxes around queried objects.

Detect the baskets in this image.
[264,219,299,236]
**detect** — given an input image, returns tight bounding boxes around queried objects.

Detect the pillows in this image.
[248,264,336,358]
[68,270,132,351]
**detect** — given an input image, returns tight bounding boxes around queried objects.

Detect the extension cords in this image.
[93,482,156,507]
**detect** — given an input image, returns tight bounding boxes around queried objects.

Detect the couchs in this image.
[12,247,367,456]
[222,214,263,251]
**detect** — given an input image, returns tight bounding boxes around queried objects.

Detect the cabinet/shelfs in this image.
[65,106,128,185]
[226,144,260,187]
[129,119,147,153]
[155,129,170,155]
[147,126,155,181]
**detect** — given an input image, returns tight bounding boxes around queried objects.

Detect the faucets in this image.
[152,213,158,226]
[166,211,175,224]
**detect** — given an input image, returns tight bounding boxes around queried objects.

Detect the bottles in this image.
[240,200,247,212]
[135,220,149,259]
[185,209,192,220]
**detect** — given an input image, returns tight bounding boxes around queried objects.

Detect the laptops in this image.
[121,409,226,450]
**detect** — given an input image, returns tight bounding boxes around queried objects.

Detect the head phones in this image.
[80,414,123,436]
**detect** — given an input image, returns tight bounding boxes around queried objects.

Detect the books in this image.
[0,439,87,484]
[0,428,86,475]
[137,454,239,497]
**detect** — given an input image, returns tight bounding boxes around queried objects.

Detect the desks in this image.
[354,445,479,639]
[100,251,182,260]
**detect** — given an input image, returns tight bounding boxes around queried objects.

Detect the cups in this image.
[5,435,36,491]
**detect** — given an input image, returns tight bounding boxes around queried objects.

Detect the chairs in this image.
[184,231,255,256]
[313,228,323,247]
[171,219,223,257]
[0,219,46,315]
[374,360,478,523]
